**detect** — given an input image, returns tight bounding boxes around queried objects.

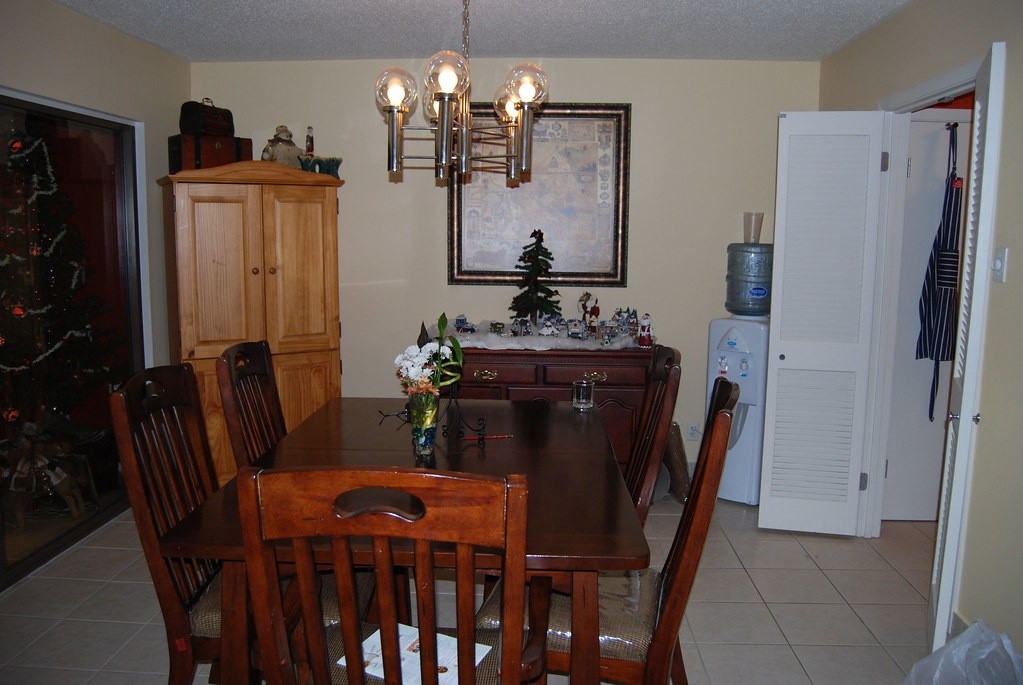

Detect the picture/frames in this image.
[447,101,633,291]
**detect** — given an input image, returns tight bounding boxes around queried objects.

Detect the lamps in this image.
[373,0,549,181]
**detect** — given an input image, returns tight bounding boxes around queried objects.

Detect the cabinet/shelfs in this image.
[156,161,345,487]
[431,348,652,477]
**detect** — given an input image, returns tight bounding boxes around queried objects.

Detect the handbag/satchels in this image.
[179,98,235,135]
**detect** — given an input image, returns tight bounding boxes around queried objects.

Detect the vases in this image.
[408,391,440,456]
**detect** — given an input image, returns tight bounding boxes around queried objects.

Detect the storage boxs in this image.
[167,135,253,176]
[409,568,484,629]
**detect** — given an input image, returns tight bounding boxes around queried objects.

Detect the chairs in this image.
[105,360,411,685]
[213,338,289,473]
[464,372,741,685]
[476,341,683,579]
[235,463,553,685]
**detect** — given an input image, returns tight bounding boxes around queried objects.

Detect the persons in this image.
[638,313,654,350]
[261,125,302,171]
[306,126,314,158]
[578,287,600,336]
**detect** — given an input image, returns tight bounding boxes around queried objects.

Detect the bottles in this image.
[725,242,773,316]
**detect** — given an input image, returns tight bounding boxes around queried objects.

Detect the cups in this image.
[571,380,594,416]
[743,211,764,244]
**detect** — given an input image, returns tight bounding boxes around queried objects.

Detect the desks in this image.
[173,399,652,685]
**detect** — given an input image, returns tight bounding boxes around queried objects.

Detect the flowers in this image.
[392,310,463,433]
